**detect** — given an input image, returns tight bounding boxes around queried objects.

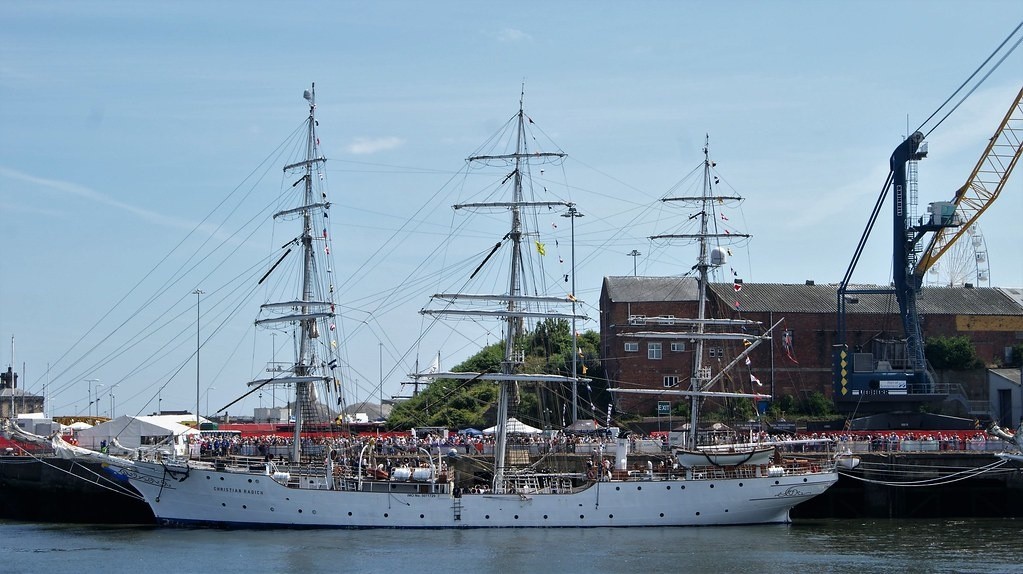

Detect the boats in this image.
[829,461,1023,519]
[839,454,860,470]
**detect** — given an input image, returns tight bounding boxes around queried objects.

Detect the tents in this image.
[456,417,608,433]
[671,423,736,447]
[59,416,213,460]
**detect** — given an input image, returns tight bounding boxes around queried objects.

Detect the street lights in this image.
[110,385,119,419]
[626,249,641,277]
[159,386,163,415]
[95,384,104,417]
[206,387,216,419]
[192,288,206,430]
[109,393,116,419]
[82,379,99,416]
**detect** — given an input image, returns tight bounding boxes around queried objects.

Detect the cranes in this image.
[831,18,1023,417]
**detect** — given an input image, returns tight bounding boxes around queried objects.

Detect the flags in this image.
[529,120,599,428]
[710,161,799,416]
[305,90,343,428]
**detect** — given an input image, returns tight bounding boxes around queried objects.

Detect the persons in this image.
[628,427,1018,454]
[142,433,614,497]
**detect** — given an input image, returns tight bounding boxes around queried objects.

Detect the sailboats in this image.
[0,75,860,532]
